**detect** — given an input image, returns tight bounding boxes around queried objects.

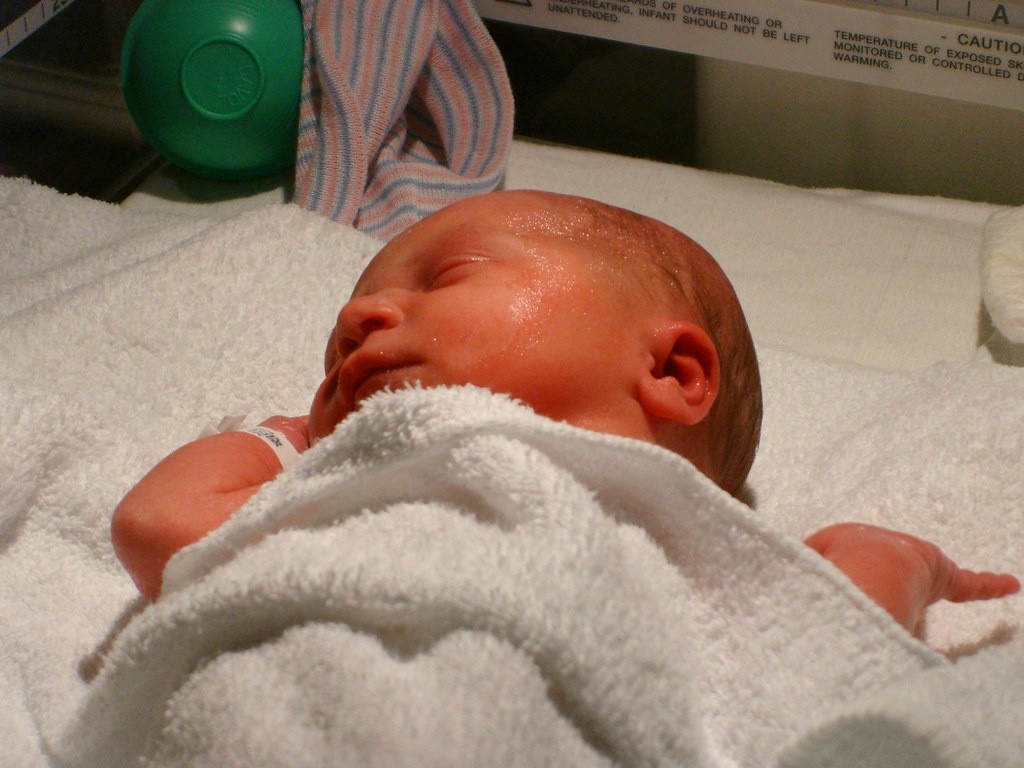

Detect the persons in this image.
[109,190,1021,768]
